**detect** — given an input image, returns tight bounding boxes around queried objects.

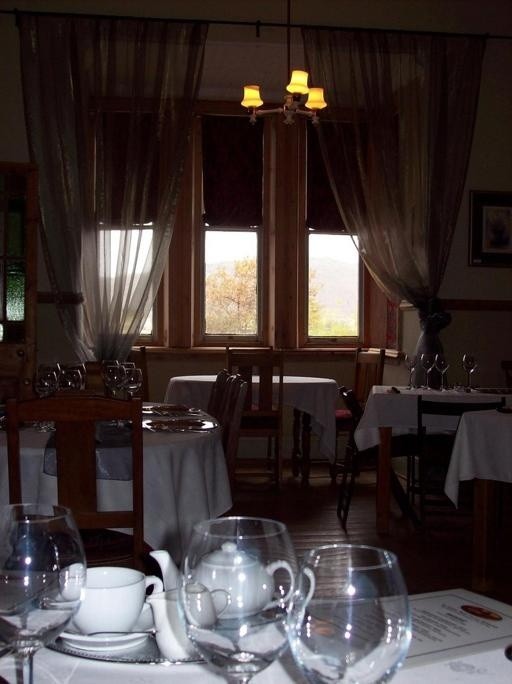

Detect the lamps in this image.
[240,0,327,128]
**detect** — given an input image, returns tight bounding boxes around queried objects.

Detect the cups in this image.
[147,584,232,660]
[69,562,165,635]
[289,544,413,683]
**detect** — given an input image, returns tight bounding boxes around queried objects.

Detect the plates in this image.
[55,605,149,651]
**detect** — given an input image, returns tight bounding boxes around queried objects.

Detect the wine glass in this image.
[405,353,418,390]
[32,360,86,432]
[101,360,144,429]
[435,353,451,391]
[0,502,88,682]
[462,352,478,392]
[420,353,435,390]
[182,514,305,683]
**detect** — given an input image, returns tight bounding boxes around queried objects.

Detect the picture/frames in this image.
[466,188,511,269]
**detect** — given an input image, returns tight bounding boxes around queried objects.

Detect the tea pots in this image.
[149,543,297,618]
